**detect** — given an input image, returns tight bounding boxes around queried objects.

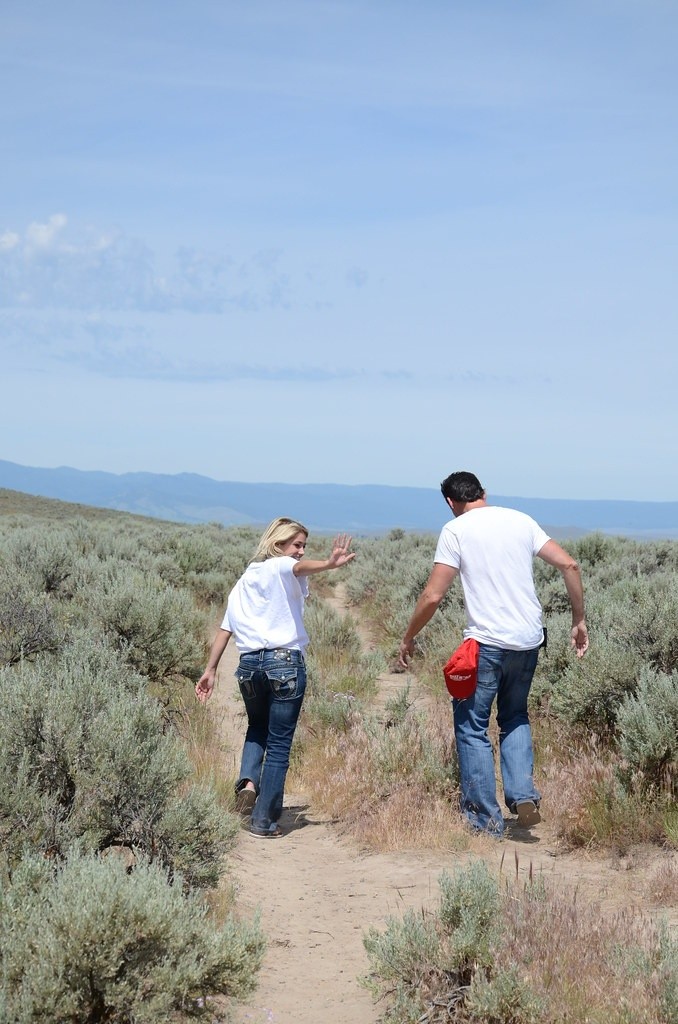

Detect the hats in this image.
[441,638,480,700]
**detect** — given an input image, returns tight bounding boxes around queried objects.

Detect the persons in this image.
[192,516,355,839]
[395,470,591,839]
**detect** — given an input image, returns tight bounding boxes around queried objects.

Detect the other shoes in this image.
[237,788,255,816]
[248,824,284,839]
[515,798,542,827]
